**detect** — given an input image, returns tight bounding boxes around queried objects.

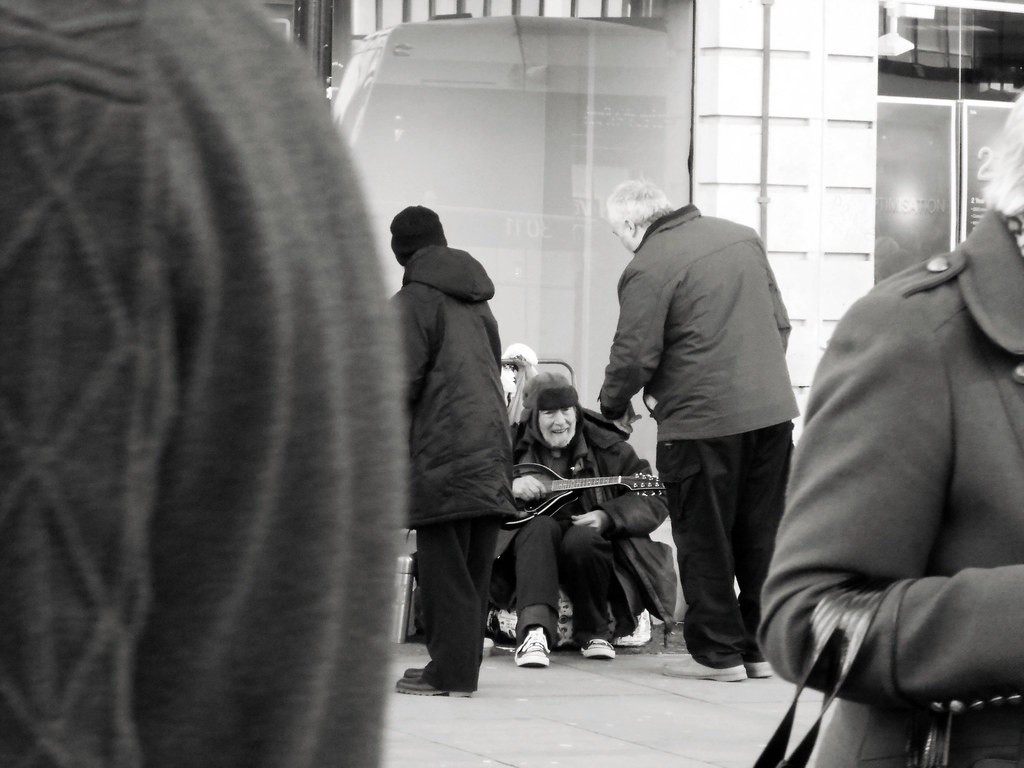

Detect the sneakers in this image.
[514,627,551,666]
[579,638,616,658]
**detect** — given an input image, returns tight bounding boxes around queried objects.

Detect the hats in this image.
[390,205,447,266]
[519,372,583,451]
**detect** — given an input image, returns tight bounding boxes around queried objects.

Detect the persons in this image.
[756,92,1024,768]
[508,372,668,668]
[0,0,408,768]
[389,206,520,698]
[597,179,799,682]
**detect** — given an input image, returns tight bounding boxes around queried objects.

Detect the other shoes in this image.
[745,656,775,678]
[662,657,748,682]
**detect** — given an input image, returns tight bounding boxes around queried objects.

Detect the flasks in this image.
[390,555,414,644]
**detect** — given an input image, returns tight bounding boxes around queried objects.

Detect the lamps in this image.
[865,8,916,57]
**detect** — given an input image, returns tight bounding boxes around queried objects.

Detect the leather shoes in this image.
[404,668,428,677]
[397,676,477,698]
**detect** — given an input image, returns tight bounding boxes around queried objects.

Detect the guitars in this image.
[499,462,666,530]
